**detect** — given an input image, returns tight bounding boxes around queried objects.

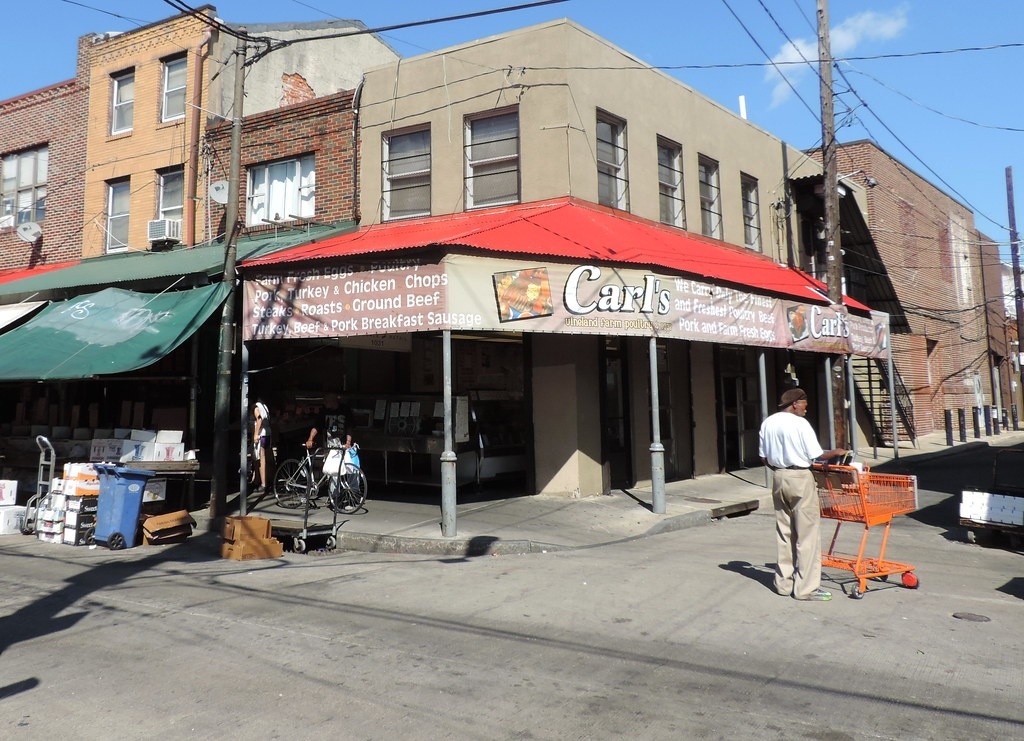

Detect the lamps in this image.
[838,170,879,188]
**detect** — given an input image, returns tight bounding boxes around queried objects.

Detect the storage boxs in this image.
[216,515,283,561]
[0,425,185,545]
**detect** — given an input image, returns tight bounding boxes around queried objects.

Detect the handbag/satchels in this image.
[344,443,360,474]
[323,448,346,476]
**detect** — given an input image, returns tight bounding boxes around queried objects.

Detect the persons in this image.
[759,389,845,600]
[306,392,360,510]
[250,390,272,490]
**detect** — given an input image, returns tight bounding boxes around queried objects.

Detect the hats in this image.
[777,389,806,406]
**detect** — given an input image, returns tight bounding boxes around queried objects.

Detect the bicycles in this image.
[273,441,368,514]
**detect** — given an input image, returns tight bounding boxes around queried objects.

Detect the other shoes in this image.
[345,505,353,510]
[252,485,265,492]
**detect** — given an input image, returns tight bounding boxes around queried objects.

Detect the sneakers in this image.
[805,588,832,601]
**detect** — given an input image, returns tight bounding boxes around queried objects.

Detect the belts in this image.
[774,465,806,470]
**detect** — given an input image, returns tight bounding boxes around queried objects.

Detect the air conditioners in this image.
[147,220,182,242]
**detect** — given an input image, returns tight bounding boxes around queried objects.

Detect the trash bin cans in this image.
[84,462,156,549]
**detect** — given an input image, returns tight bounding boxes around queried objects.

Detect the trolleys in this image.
[958,448,1024,553]
[809,450,919,600]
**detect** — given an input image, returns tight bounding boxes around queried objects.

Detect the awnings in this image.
[0,284,229,380]
